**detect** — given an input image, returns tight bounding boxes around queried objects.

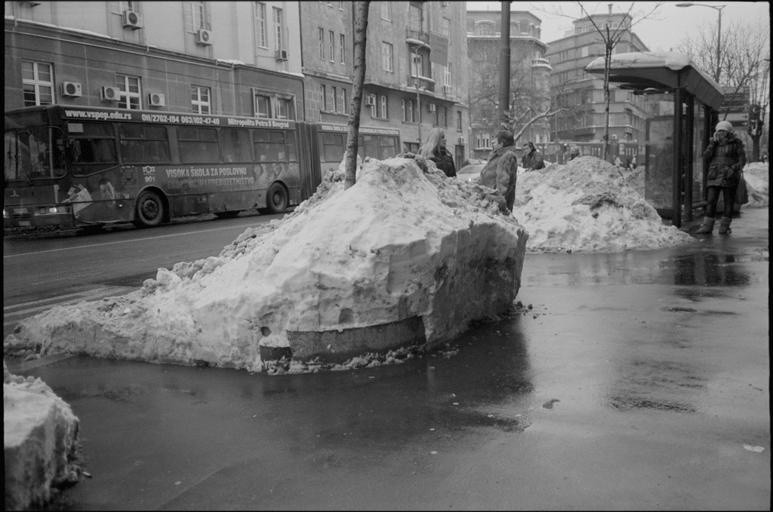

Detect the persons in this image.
[695,121,747,235]
[418,128,457,177]
[66,180,133,221]
[479,130,515,211]
[522,142,544,171]
[253,151,301,189]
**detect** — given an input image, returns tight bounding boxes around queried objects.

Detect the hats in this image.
[715,121,733,133]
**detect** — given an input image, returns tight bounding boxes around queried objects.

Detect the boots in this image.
[702,217,715,233]
[719,217,731,233]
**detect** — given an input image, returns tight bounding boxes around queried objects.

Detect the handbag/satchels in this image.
[735,181,748,204]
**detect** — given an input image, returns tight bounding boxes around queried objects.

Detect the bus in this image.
[3,104,403,242]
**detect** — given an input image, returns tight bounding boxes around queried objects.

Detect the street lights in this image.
[677,3,727,84]
